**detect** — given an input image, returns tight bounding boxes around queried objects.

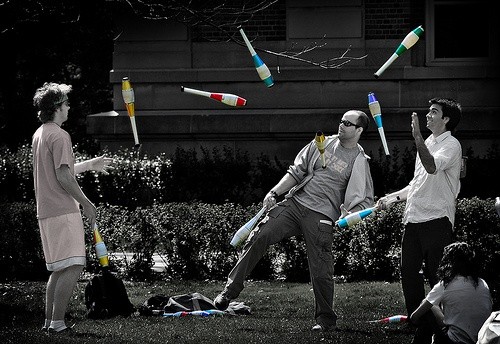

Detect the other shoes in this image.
[213,290,231,310]
[311,323,337,332]
[43,326,75,336]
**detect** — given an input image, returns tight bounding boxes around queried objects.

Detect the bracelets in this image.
[270,189,278,197]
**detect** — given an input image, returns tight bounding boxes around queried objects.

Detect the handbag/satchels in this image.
[138,293,213,318]
[85,271,136,318]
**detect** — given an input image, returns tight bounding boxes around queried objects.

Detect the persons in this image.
[213,109,374,332]
[32,81,115,337]
[376,96,462,316]
[406,241,492,344]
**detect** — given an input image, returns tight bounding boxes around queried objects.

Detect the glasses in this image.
[339,119,357,127]
